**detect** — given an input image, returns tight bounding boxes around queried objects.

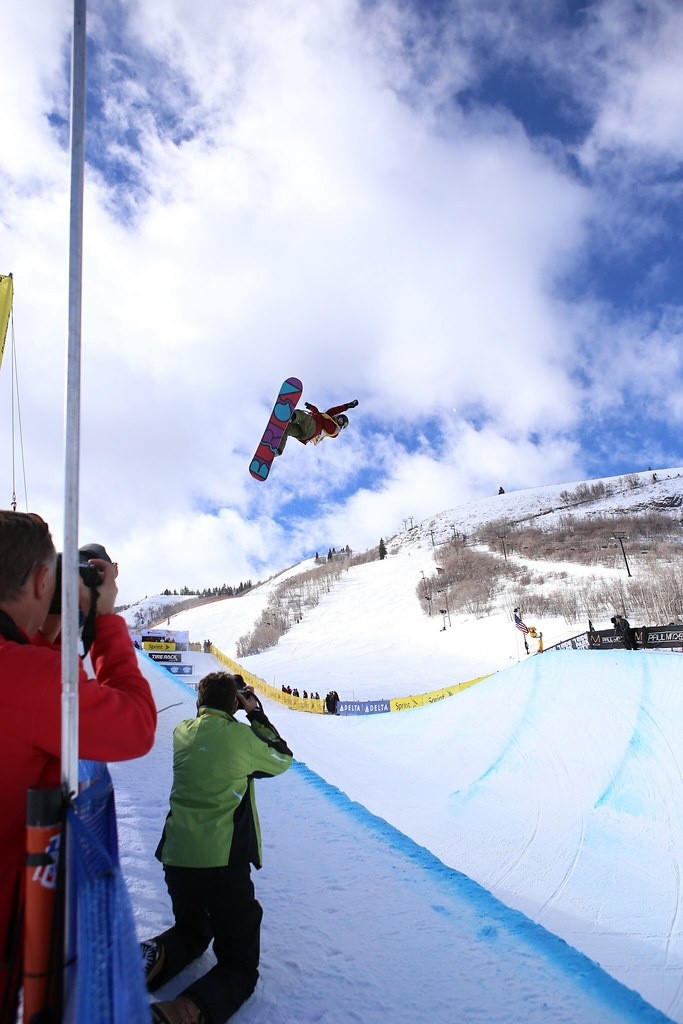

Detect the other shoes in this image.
[138,939,163,985]
[148,996,203,1024]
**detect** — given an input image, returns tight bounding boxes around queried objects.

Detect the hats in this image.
[0,510,47,583]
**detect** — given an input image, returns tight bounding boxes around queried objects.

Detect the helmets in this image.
[337,414,349,429]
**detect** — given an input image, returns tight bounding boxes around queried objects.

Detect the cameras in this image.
[48,544,117,616]
[233,674,249,710]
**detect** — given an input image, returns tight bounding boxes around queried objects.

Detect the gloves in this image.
[305,402,312,409]
[348,400,358,408]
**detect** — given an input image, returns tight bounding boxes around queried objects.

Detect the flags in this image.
[515,614,529,634]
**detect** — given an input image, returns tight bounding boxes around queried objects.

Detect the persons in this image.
[282,685,339,715]
[274,399,359,457]
[138,672,293,1024]
[614,615,649,650]
[134,637,213,653]
[0,511,158,1024]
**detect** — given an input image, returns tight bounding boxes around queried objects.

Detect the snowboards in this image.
[249,377,303,482]
